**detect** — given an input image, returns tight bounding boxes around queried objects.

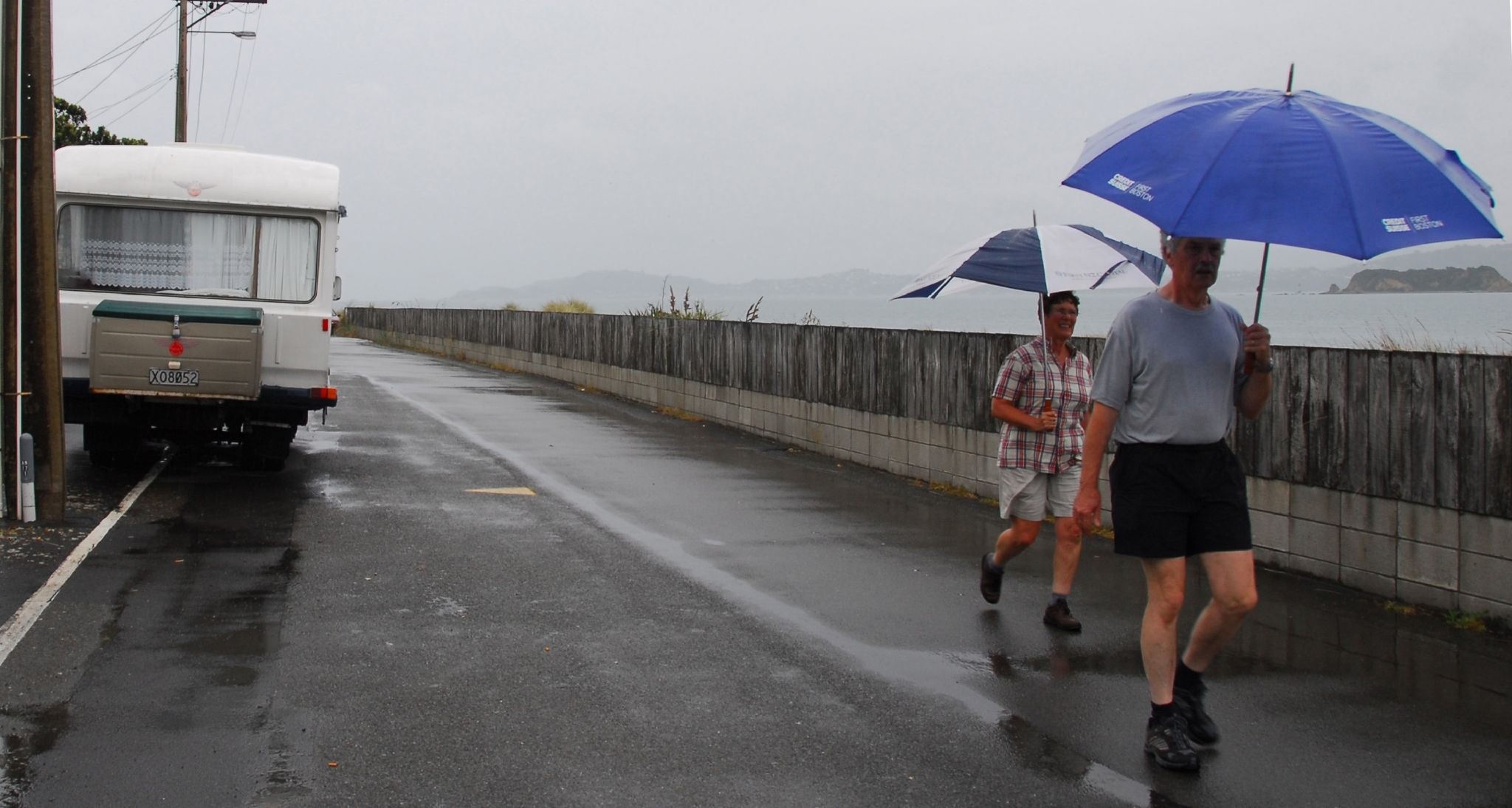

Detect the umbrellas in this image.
[888,207,1167,432]
[1061,63,1505,368]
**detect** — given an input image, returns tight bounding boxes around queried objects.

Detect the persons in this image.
[980,289,1093,632]
[1072,228,1274,771]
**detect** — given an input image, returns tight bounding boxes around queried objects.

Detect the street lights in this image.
[174,26,257,140]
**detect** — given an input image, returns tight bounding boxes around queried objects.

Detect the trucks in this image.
[53,144,343,468]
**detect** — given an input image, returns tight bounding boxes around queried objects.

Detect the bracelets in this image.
[1254,358,1274,373]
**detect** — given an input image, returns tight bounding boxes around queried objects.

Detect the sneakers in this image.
[1144,717,1201,777]
[1174,689,1220,753]
[1043,598,1081,633]
[980,552,1004,603]
